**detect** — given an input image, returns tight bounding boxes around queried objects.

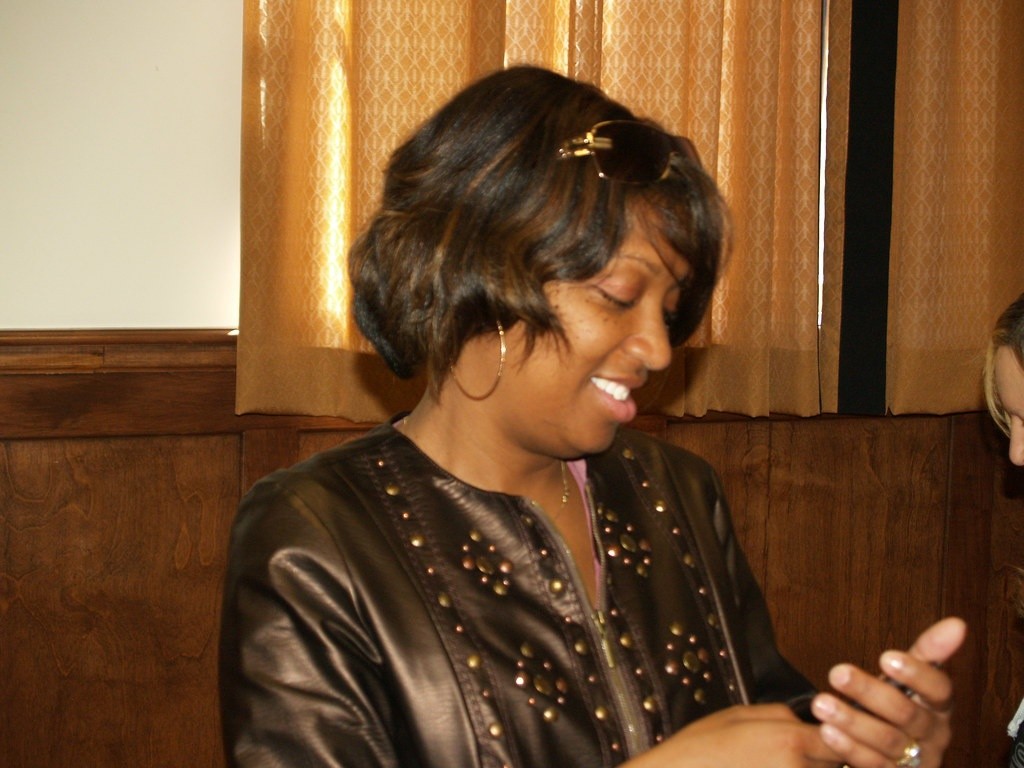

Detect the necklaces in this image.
[396,408,572,534]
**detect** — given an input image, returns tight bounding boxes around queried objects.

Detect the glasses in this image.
[555,121,702,188]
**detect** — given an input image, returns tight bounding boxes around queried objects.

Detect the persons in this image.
[209,58,967,768]
[978,289,1024,768]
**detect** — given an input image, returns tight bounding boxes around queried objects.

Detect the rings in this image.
[894,736,924,768]
